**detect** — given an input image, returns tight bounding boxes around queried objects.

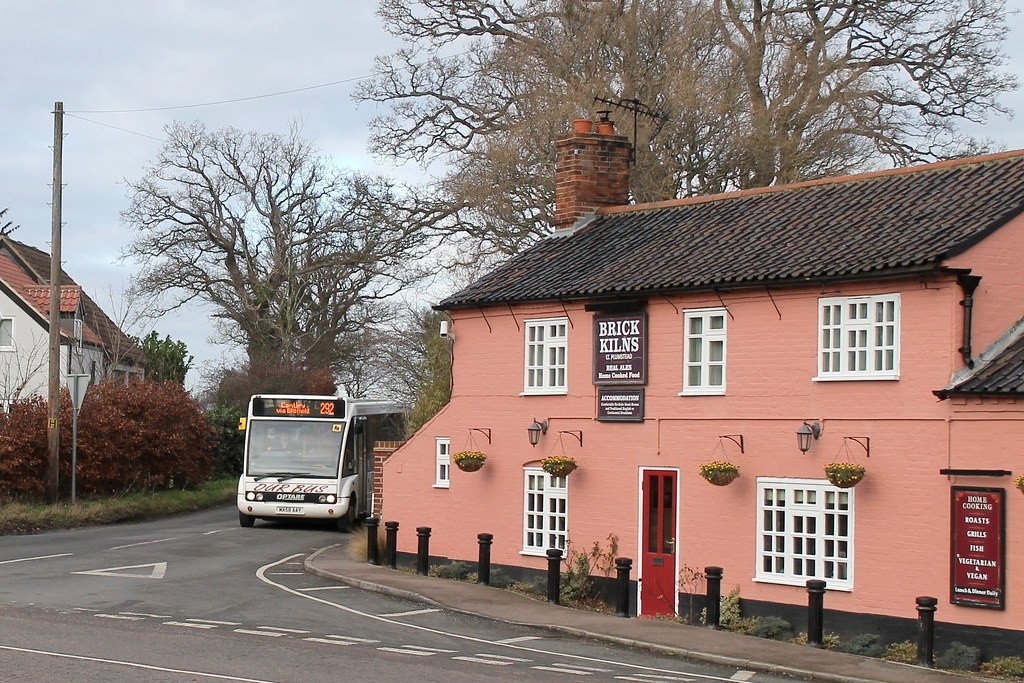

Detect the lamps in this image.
[527,418,547,447]
[797,419,821,454]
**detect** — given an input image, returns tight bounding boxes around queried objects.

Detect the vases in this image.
[706,471,737,485]
[574,120,593,133]
[548,466,576,476]
[457,458,482,473]
[831,473,862,488]
[596,120,615,134]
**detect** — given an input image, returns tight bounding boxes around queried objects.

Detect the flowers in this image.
[824,463,866,484]
[540,455,578,477]
[452,452,487,468]
[697,462,740,480]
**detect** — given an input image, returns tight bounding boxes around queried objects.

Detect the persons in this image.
[267,430,296,454]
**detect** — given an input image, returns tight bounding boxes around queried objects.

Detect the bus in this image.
[236,392,411,530]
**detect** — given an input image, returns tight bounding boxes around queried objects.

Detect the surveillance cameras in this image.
[439,321,448,338]
